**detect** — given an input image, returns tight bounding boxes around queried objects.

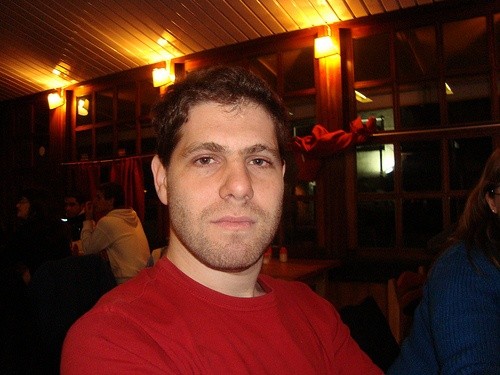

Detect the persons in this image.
[0,180,153,289]
[59,64,384,375]
[384,146,500,375]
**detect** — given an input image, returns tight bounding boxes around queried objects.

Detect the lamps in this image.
[152,68,173,87]
[314,36,339,59]
[47,92,64,109]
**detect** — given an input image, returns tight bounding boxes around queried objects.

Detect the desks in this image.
[261,258,340,300]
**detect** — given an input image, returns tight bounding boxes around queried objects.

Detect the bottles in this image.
[279,247,288,263]
[263,247,273,265]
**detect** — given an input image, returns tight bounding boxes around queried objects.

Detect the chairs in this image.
[19,253,117,375]
[337,295,402,375]
[386,279,406,349]
